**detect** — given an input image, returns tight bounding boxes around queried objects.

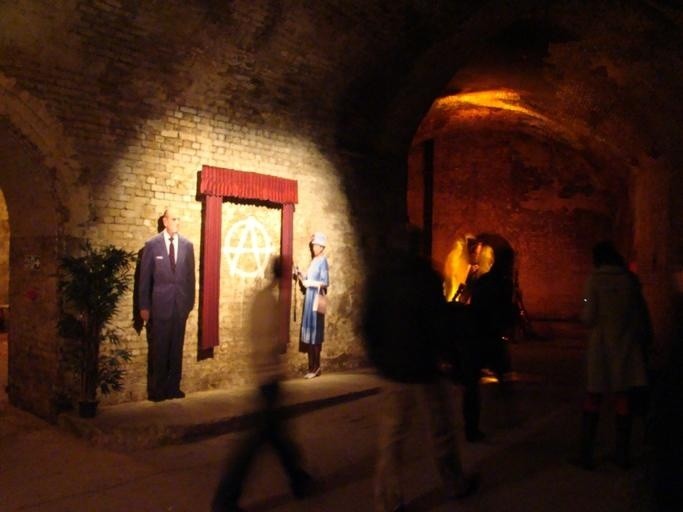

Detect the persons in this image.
[136,207,196,400]
[296,231,330,379]
[362,225,479,512]
[563,247,657,468]
[460,235,518,441]
[209,255,318,511]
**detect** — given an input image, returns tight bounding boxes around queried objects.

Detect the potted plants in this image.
[57,249,139,418]
